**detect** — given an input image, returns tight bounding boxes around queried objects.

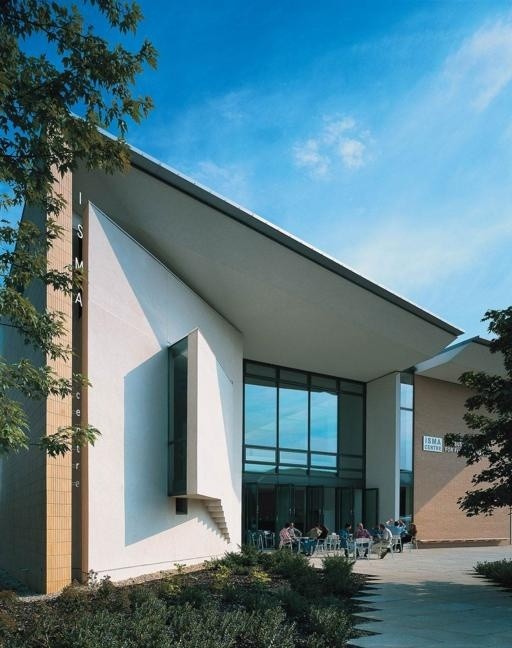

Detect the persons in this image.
[279,518,417,559]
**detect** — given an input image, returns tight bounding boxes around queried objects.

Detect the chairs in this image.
[249,530,402,561]
